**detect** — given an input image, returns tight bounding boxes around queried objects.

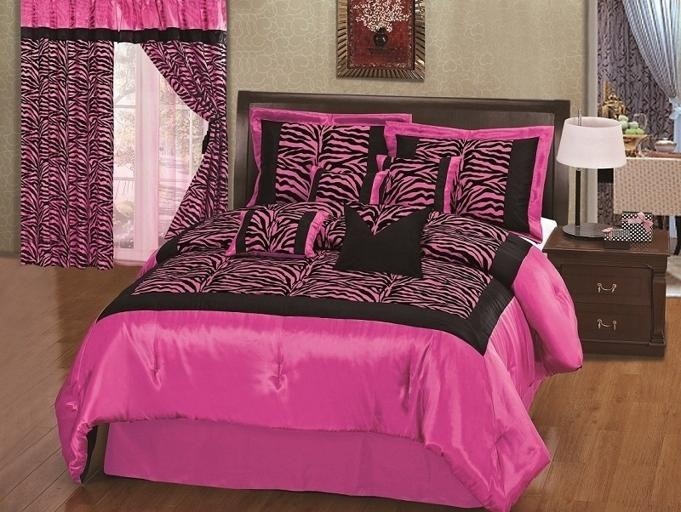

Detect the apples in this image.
[624,128,643,134]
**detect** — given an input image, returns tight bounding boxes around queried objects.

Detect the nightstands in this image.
[542,222,673,359]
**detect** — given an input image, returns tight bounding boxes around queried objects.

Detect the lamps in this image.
[556,114,628,241]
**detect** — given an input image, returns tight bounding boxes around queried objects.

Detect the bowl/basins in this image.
[654,144,677,154]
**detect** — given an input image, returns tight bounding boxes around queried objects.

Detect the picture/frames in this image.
[336,1,425,81]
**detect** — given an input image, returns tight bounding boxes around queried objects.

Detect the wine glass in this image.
[634,114,647,158]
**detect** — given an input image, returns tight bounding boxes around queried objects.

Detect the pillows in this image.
[333,202,437,279]
[309,165,391,208]
[376,154,463,216]
[246,106,413,206]
[384,118,558,245]
[226,208,330,262]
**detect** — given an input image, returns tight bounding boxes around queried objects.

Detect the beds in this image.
[76,89,571,511]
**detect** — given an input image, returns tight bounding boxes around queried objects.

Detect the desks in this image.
[608,151,681,256]
